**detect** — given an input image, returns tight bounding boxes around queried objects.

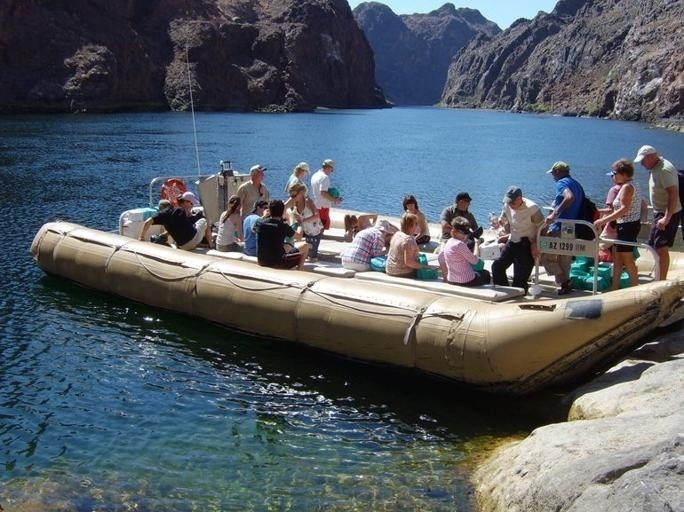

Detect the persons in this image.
[492,145,683,292]
[342,191,491,288]
[142,159,341,271]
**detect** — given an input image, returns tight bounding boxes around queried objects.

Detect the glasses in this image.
[291,192,299,198]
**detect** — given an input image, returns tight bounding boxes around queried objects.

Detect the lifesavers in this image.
[416,267,441,281]
[472,256,484,272]
[568,256,632,291]
[369,253,429,273]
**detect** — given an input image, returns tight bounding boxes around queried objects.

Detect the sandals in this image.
[559,279,574,295]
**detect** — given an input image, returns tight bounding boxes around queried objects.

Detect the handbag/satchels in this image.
[599,222,617,250]
[576,196,600,240]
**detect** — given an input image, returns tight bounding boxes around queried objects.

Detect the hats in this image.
[228,195,240,205]
[249,165,267,173]
[633,144,657,163]
[454,223,472,234]
[297,162,310,175]
[182,192,200,205]
[323,160,335,169]
[455,192,472,203]
[503,188,522,203]
[546,162,569,174]
[373,220,394,234]
[251,201,268,213]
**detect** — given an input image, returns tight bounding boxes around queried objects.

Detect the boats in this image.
[31,174,684,398]
